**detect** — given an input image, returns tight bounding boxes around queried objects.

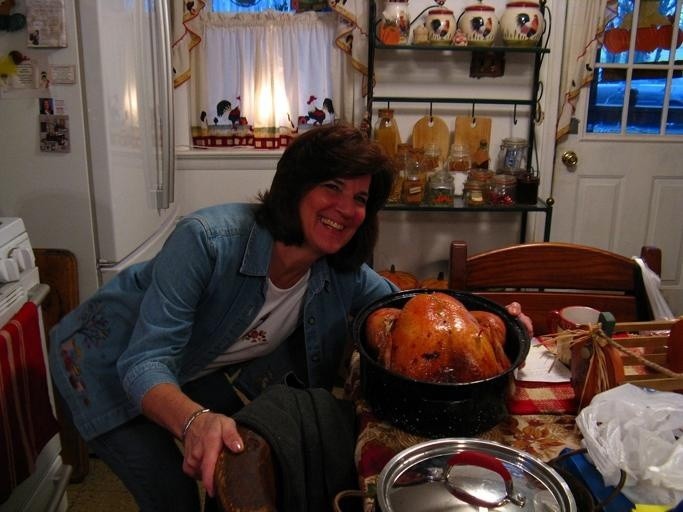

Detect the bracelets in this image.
[181,406,209,439]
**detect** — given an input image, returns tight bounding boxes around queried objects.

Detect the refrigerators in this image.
[1,0,181,303]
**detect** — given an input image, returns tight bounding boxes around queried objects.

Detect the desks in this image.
[374,196,553,251]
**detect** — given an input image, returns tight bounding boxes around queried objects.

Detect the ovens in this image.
[0,267,73,512]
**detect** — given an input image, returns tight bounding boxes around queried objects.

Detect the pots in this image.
[336,438,577,512]
[353,288,531,439]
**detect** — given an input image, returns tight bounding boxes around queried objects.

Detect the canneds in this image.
[403,177,422,204]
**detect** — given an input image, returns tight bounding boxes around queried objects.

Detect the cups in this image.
[545,306,601,364]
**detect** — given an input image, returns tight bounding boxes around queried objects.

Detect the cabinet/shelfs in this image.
[364,0,551,200]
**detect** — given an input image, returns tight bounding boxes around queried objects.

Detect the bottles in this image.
[380,1,546,49]
[371,108,539,205]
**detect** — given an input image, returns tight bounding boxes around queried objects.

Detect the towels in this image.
[0,301,60,504]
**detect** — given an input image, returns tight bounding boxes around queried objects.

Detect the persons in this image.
[41,99,54,115]
[43,119,534,512]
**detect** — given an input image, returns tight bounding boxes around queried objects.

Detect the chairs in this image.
[448,240,662,331]
[43,276,277,510]
